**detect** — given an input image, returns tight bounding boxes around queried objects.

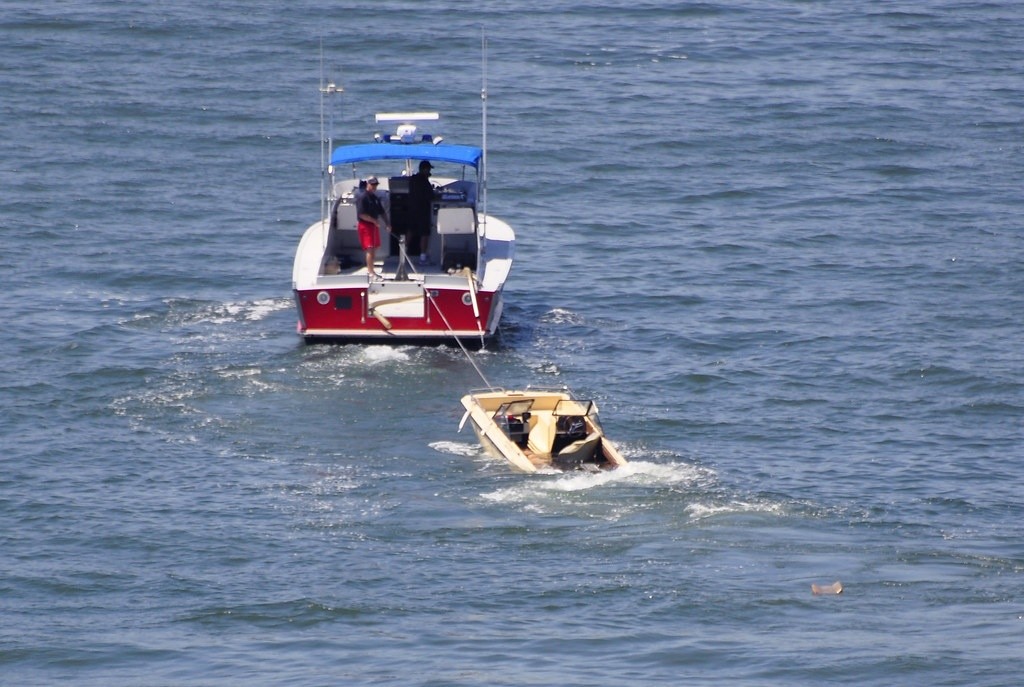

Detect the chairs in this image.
[527,396,592,451]
[336,203,360,231]
[438,207,476,266]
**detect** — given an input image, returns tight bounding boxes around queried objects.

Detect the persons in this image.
[408,159,445,266]
[355,176,393,277]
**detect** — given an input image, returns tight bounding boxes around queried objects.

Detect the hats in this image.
[368,176,381,185]
[418,160,435,169]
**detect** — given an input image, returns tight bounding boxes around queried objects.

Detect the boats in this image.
[291,111,515,350]
[461,387,628,477]
